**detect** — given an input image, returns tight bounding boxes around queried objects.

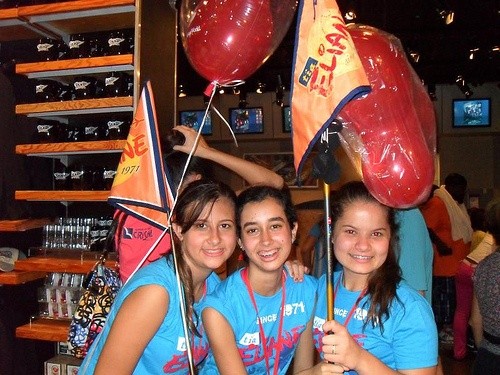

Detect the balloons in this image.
[333,22,437,209]
[178,0,299,97]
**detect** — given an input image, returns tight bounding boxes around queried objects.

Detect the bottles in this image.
[36,271,100,321]
[42,215,122,254]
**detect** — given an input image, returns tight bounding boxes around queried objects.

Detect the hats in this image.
[0,248,27,272]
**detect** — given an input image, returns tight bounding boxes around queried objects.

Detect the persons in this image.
[76,125,498,375]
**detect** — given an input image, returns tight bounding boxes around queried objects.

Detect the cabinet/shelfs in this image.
[0,0,177,375]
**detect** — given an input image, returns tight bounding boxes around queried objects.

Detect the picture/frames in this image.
[242,151,320,190]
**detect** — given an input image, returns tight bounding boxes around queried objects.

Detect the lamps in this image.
[440,9,456,26]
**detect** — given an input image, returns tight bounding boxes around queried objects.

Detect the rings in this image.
[331,345,336,354]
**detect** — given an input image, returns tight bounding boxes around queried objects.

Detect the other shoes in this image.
[438,330,454,344]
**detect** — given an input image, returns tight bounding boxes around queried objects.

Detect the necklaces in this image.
[332,274,368,327]
[186,278,207,369]
[243,267,286,375]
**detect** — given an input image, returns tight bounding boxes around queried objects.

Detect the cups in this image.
[33,118,132,143]
[32,70,134,104]
[54,162,117,191]
[34,30,135,60]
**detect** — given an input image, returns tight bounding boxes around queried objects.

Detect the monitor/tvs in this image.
[282,106,291,133]
[229,107,264,134]
[452,99,491,127]
[180,110,213,136]
